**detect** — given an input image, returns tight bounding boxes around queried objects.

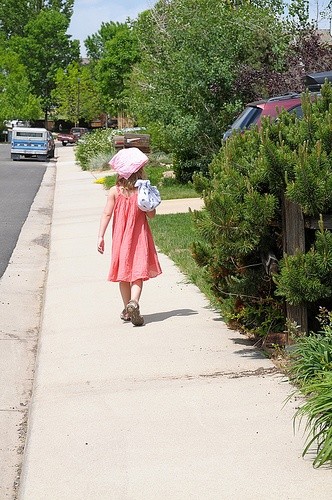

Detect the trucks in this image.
[10,127,55,162]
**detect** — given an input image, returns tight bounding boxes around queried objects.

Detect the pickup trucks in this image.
[57,127,89,146]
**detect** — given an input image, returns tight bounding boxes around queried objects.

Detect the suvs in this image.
[222,93,332,144]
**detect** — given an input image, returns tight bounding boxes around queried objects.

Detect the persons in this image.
[97,147,163,326]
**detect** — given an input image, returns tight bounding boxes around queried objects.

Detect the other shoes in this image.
[120,309,130,320]
[126,300,144,326]
